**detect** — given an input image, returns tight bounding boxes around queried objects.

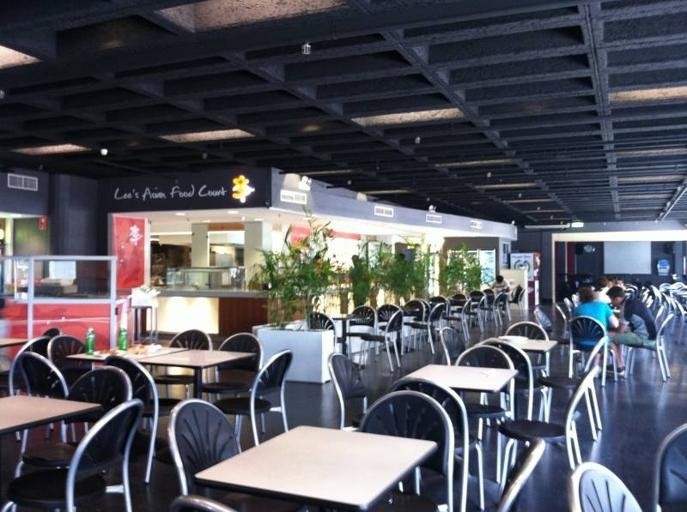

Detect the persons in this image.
[394,253,411,306]
[491,276,512,316]
[569,272,662,376]
[153,255,167,276]
[312,256,323,280]
[350,255,368,308]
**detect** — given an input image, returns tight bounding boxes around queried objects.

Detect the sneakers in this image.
[607,365,622,376]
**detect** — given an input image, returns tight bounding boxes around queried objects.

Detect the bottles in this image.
[118,327,128,349]
[85,327,95,355]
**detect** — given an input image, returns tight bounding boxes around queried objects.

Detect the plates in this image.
[144,343,161,353]
[498,335,528,341]
[91,349,127,358]
